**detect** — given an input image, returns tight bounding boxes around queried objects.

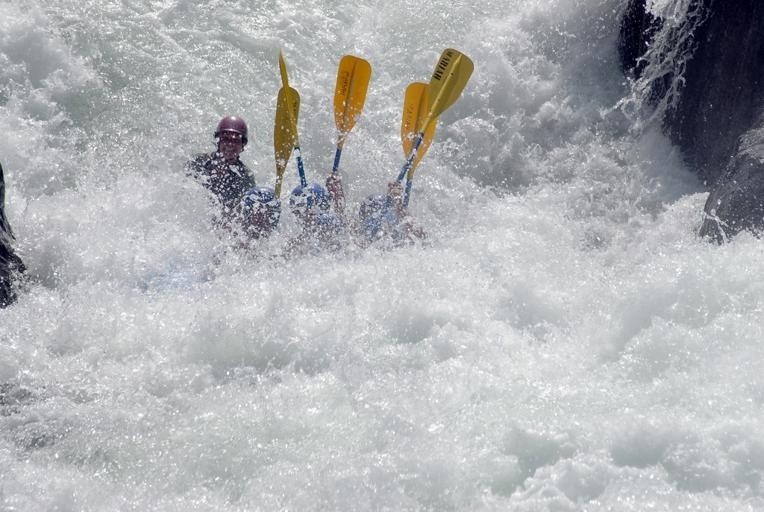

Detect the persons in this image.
[225,172,426,258]
[187,115,256,203]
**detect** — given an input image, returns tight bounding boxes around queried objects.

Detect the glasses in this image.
[220,136,241,143]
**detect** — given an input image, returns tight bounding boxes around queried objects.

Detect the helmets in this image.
[289,183,330,219]
[243,186,280,226]
[359,193,385,219]
[215,115,247,139]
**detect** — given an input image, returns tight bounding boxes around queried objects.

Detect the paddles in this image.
[279,49,307,187]
[386,47,474,203]
[274,86,300,199]
[400,83,439,210]
[332,55,371,174]
